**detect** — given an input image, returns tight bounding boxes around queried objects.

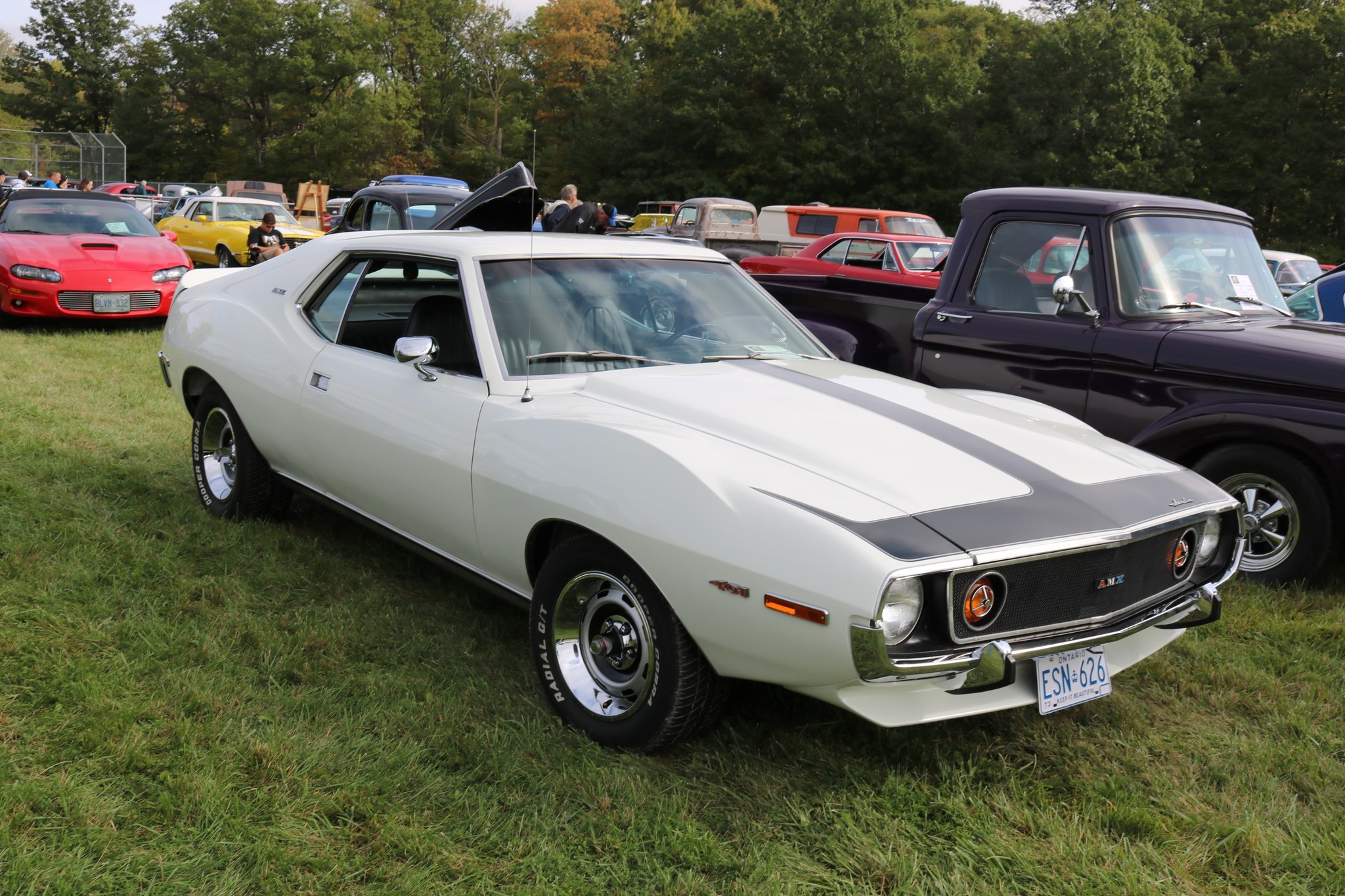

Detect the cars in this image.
[325,174,858,365]
[0,177,328,268]
[157,226,1249,758]
[739,231,1344,326]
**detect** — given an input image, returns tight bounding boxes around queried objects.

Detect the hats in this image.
[602,203,617,226]
[18,170,33,179]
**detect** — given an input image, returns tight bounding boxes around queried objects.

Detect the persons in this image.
[44,171,61,188]
[134,180,149,199]
[541,184,577,233]
[0,168,6,205]
[78,177,93,192]
[247,212,288,263]
[56,175,68,188]
[11,170,33,188]
[551,203,617,235]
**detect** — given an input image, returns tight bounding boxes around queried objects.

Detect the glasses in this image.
[266,224,276,228]
[574,194,578,197]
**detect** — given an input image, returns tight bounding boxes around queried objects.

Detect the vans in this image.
[756,201,948,272]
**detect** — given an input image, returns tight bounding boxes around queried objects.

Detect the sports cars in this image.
[0,190,191,324]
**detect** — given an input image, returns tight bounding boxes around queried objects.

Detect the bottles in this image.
[252,243,258,248]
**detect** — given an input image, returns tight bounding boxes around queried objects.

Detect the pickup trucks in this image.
[641,196,811,267]
[742,186,1344,585]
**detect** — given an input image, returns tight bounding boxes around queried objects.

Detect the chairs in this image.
[244,226,261,264]
[549,292,638,373]
[1055,270,1096,313]
[976,266,1042,313]
[405,294,473,372]
